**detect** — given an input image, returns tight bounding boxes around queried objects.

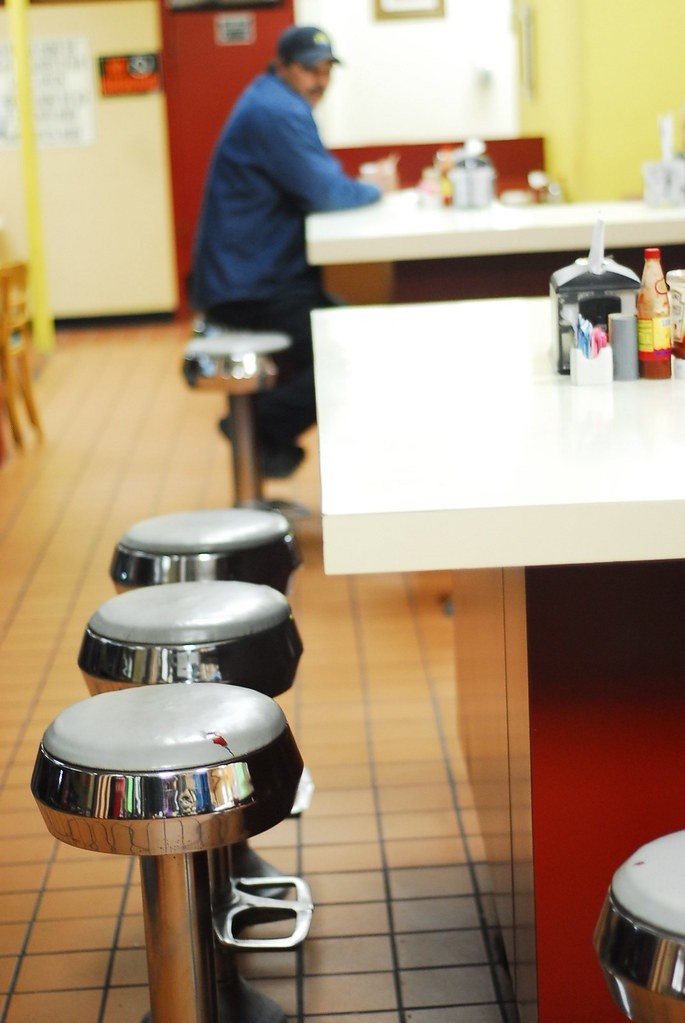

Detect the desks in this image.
[300,189,685,297]
[311,280,685,1022]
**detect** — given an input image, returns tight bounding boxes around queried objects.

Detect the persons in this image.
[189,27,380,475]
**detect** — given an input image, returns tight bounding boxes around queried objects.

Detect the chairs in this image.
[2,262,49,452]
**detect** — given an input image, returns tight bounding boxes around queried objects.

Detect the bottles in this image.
[667,269,685,360]
[637,248,674,379]
[417,169,443,209]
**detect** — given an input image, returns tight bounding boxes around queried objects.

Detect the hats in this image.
[278,27,341,71]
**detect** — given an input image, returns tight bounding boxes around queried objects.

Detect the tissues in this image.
[446,137,498,208]
[550,222,642,375]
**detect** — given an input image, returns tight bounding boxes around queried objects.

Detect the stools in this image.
[30,682,313,1023]
[592,831,684,1023]
[73,581,314,907]
[184,311,295,510]
[108,509,306,596]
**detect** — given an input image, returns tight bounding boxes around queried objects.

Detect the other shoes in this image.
[218,412,305,478]
